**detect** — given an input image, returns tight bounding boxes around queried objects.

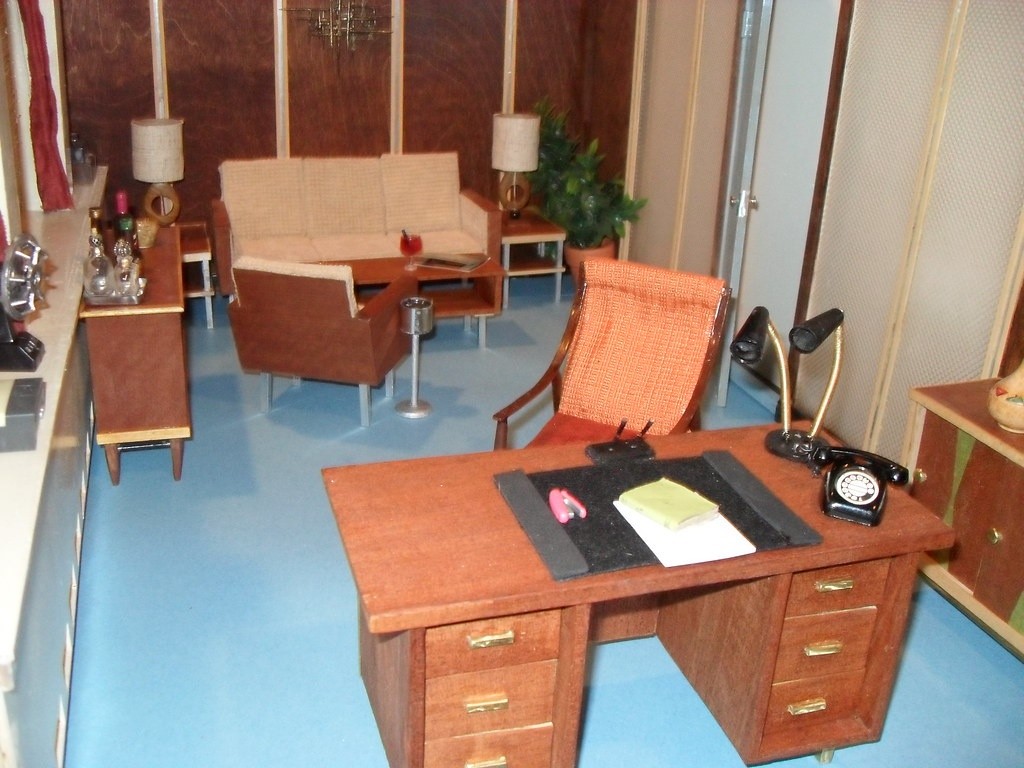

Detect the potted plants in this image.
[526,96,649,284]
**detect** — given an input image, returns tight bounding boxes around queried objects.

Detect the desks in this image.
[80,228,191,485]
[322,420,956,768]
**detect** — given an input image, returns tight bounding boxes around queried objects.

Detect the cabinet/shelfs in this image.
[909,274,1024,663]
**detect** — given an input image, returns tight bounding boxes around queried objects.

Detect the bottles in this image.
[90,206,105,255]
[84,247,139,305]
[113,192,138,259]
[70,132,84,164]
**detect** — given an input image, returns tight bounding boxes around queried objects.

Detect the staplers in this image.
[550,488,586,522]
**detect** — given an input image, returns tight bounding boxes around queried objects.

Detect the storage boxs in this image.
[72,152,96,185]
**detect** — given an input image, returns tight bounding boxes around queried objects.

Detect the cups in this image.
[135,218,160,248]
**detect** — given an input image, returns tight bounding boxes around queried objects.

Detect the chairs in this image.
[227,258,418,426]
[491,257,731,451]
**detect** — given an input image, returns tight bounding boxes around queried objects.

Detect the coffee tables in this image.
[175,224,215,328]
[495,209,566,306]
[309,253,507,348]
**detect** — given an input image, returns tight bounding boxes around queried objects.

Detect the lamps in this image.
[281,0,394,48]
[395,296,433,419]
[492,113,541,219]
[730,307,843,461]
[132,119,183,226]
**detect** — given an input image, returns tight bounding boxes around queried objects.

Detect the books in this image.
[412,251,490,272]
[619,477,719,531]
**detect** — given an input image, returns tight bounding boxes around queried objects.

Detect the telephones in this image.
[812,445,910,526]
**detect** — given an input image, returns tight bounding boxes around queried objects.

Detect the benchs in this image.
[208,152,502,303]
